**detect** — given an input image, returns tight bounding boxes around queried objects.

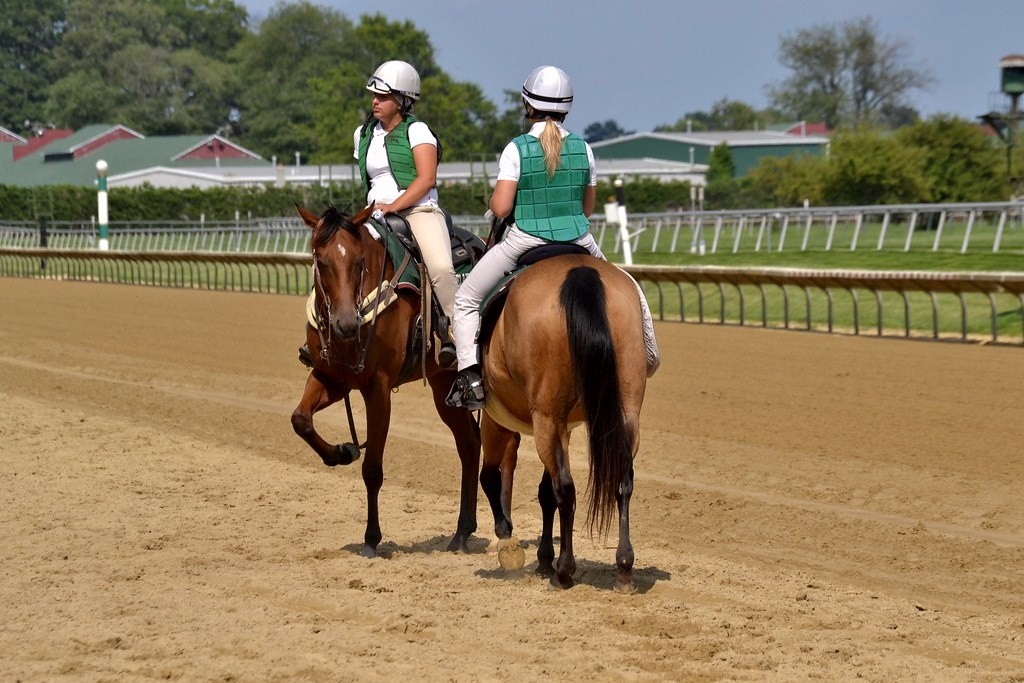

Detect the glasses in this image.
[367,75,391,96]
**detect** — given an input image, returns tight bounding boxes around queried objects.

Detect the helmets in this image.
[520,65,573,114]
[365,60,420,102]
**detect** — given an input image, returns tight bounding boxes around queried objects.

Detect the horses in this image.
[290,200,520,558]
[480,218,648,593]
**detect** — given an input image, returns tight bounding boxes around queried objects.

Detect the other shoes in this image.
[446,367,485,411]
[439,344,457,365]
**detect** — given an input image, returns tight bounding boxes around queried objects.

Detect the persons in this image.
[444,66,608,411]
[299,60,459,369]
